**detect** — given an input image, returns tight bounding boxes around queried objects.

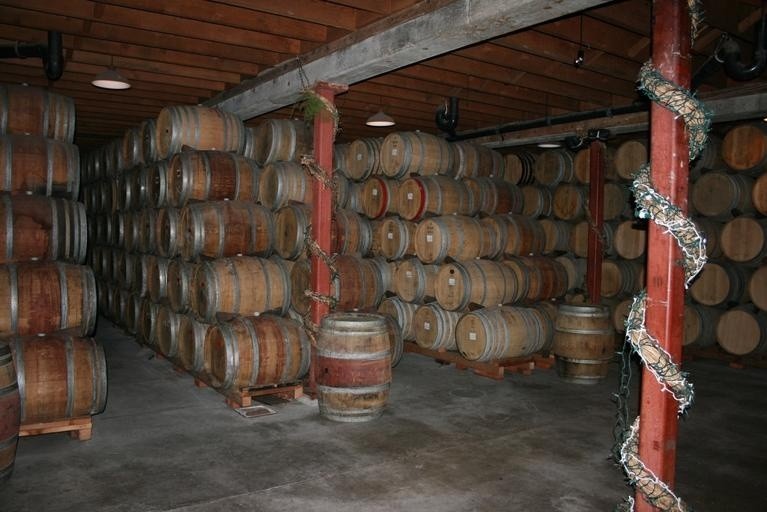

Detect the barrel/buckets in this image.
[1,81,766,490]
